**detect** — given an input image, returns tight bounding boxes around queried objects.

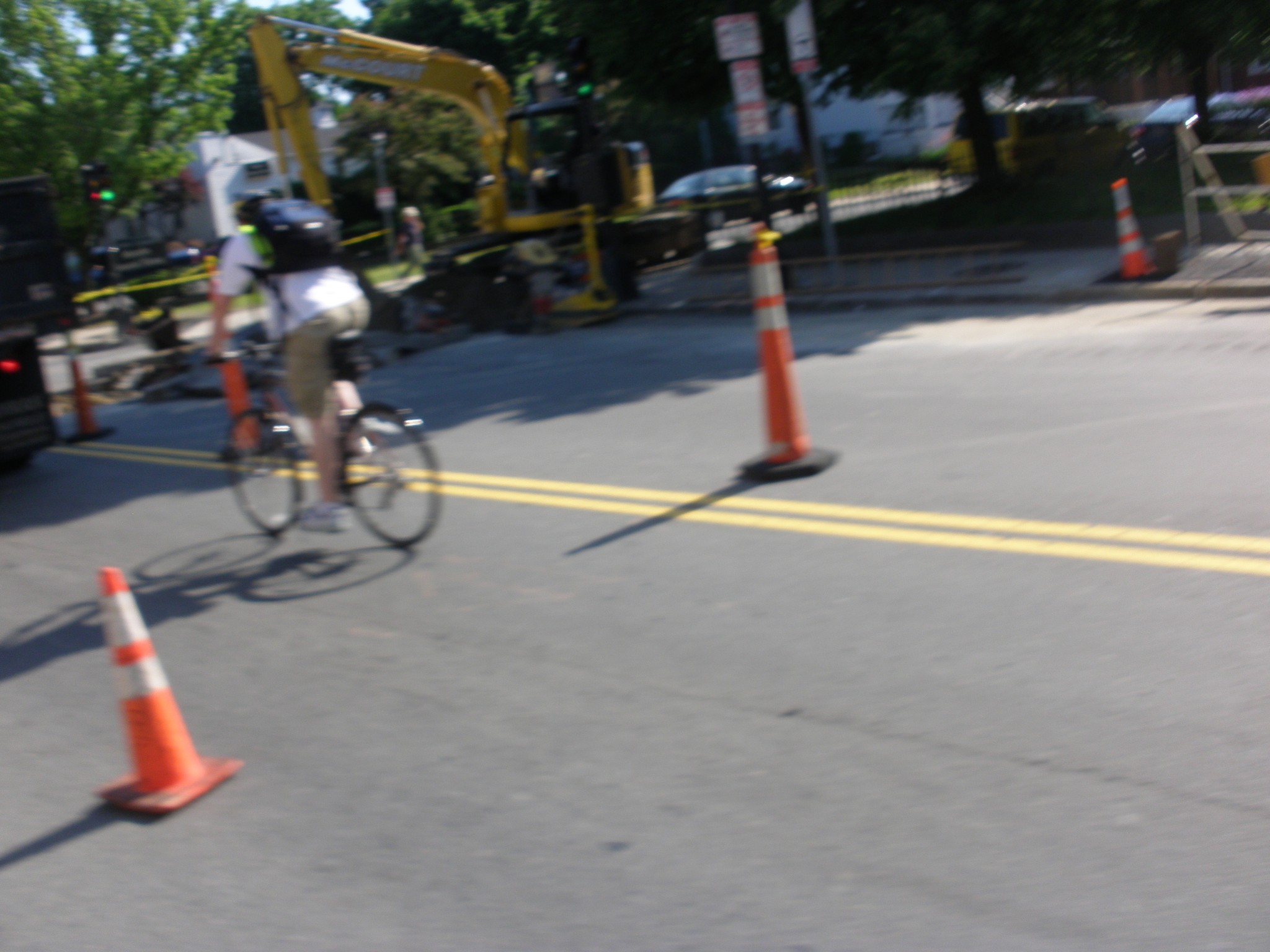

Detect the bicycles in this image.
[204,332,446,550]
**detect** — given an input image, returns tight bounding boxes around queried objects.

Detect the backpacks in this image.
[241,196,347,274]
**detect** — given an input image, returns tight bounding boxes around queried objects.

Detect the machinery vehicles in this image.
[245,14,724,339]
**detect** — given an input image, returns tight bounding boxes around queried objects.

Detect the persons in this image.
[206,158,372,533]
[395,205,432,279]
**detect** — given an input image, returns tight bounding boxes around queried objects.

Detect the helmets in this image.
[228,189,266,220]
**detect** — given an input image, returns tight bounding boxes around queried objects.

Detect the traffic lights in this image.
[79,162,117,209]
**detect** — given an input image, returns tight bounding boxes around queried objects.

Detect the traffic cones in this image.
[93,564,243,816]
[1093,176,1181,283]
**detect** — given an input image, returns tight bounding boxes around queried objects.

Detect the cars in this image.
[940,77,1269,187]
[0,238,207,473]
[652,164,817,235]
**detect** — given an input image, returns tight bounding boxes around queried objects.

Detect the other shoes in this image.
[341,437,370,457]
[300,506,352,534]
